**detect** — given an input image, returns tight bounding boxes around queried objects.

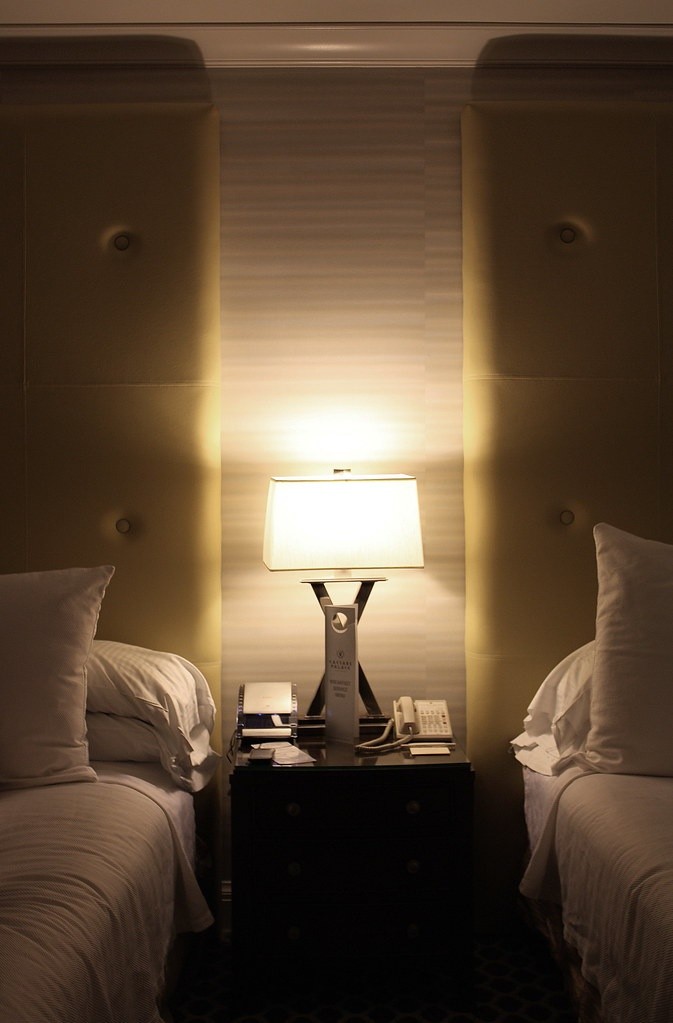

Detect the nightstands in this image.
[226,732,473,993]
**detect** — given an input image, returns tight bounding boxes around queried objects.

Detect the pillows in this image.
[570,522,673,777]
[84,713,223,792]
[0,565,117,791]
[86,639,216,768]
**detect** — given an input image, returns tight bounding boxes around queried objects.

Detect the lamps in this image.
[262,467,424,737]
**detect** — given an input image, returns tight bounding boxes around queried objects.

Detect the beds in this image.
[460,32,673,1023]
[0,37,241,1023]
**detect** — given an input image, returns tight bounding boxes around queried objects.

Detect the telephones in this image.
[393,695,452,742]
